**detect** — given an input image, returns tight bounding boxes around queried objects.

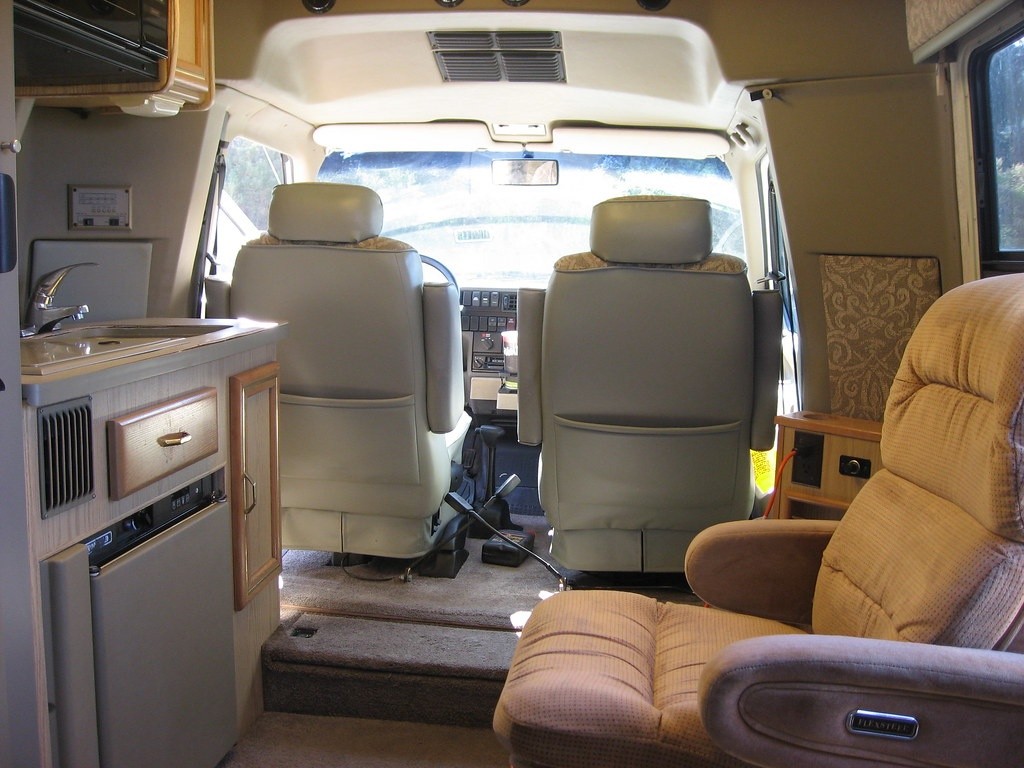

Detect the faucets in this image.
[25,260,101,333]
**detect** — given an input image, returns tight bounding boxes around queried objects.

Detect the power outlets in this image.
[791,430,824,489]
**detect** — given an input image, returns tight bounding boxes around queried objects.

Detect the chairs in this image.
[494,271,1024,768]
[517,196,783,591]
[205,182,475,578]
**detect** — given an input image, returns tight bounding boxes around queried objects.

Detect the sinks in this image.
[65,318,236,347]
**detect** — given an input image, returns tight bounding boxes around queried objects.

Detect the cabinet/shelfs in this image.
[21,321,291,768]
